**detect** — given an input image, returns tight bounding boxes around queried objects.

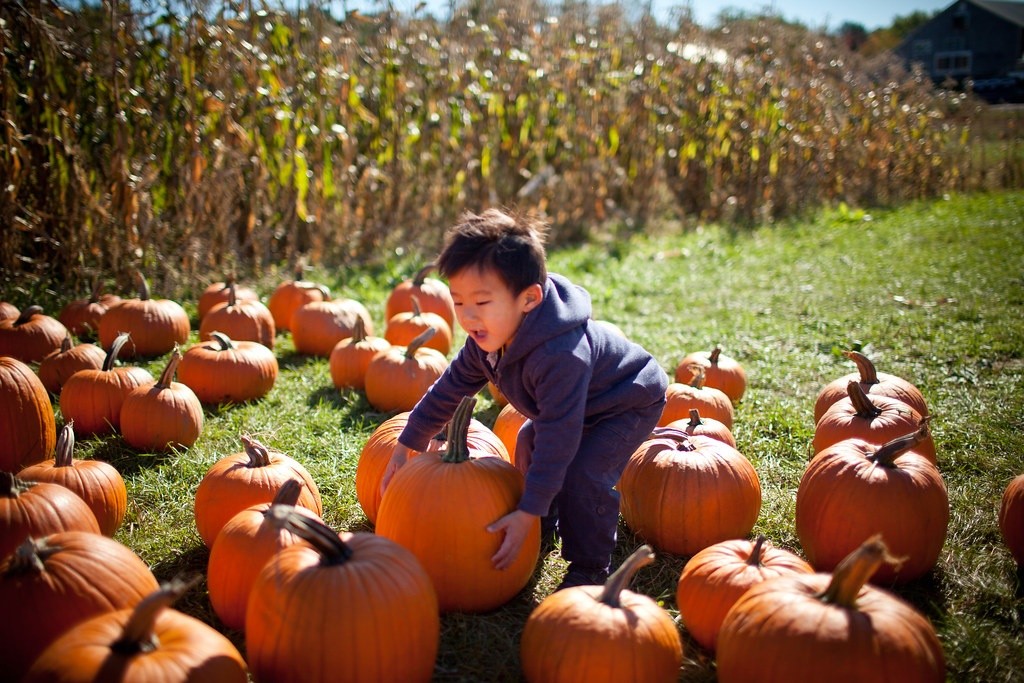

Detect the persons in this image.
[379,208,668,599]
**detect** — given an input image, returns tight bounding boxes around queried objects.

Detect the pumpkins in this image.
[521,346,948,683]
[998,474,1024,573]
[0,265,539,683]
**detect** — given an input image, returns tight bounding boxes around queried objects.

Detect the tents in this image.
[891,0,1024,106]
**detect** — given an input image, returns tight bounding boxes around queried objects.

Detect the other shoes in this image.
[553,567,608,593]
[540,518,559,557]
